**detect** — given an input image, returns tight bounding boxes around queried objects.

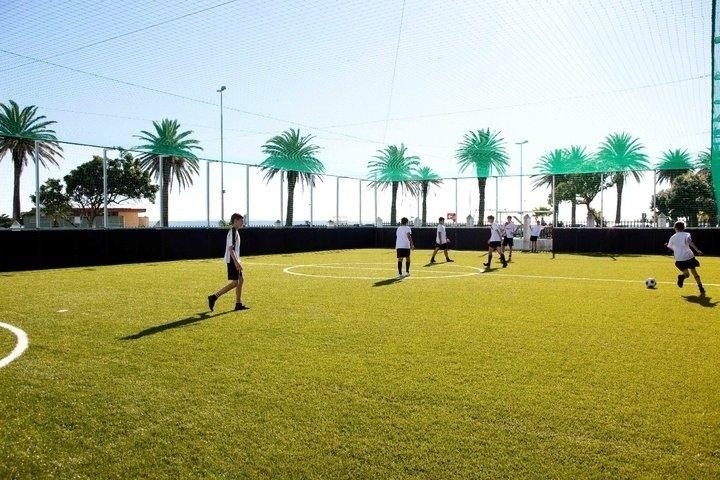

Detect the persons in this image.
[499,215,519,260]
[529,221,547,252]
[208,214,250,311]
[431,217,453,263]
[483,216,508,268]
[396,217,415,278]
[667,222,705,293]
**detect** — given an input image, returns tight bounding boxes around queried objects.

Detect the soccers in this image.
[646,278,656,289]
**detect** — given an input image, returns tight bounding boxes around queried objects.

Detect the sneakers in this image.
[208,295,217,311]
[235,303,250,309]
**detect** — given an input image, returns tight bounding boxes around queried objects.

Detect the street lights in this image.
[514,139,529,224]
[215,85,227,221]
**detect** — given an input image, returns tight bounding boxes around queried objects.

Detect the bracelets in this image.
[446,237,447,239]
